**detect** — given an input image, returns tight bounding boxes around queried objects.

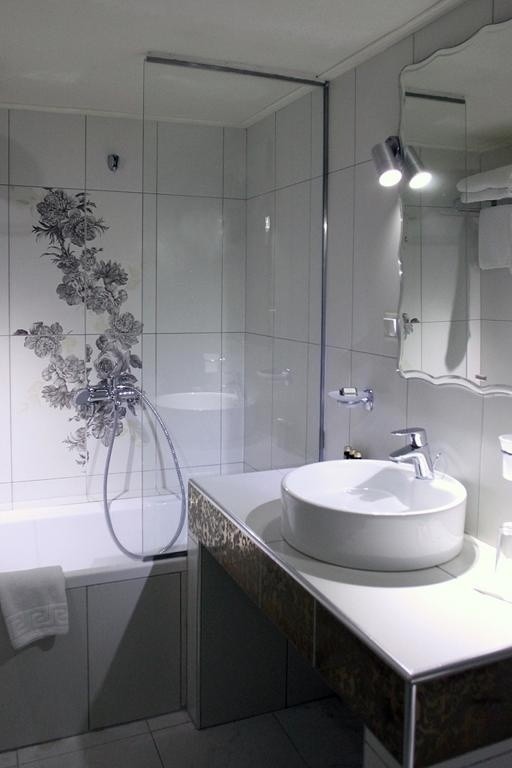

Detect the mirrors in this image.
[398,18,512,396]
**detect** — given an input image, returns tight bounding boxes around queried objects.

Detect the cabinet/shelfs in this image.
[2,557,185,752]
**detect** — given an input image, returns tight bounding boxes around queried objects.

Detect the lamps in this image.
[372,137,402,193]
[401,140,431,190]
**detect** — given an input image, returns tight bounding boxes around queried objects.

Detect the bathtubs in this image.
[1,493,186,580]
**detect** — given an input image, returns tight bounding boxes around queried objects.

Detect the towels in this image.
[458,165,512,203]
[480,204,512,269]
[2,566,70,648]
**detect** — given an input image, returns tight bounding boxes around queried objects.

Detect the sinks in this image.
[151,392,241,449]
[279,458,469,573]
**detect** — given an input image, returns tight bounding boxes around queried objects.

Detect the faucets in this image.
[205,348,247,388]
[387,428,435,482]
